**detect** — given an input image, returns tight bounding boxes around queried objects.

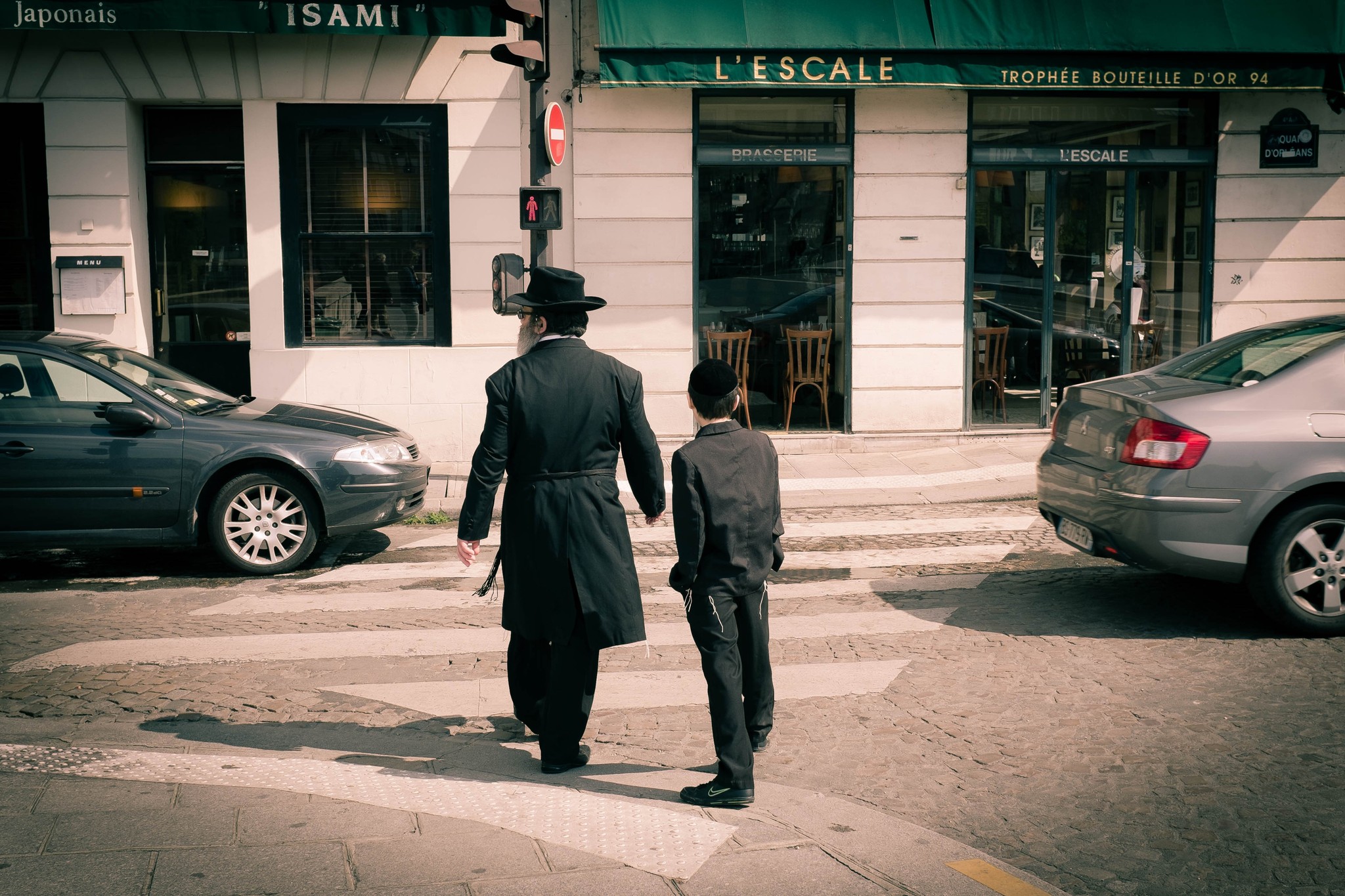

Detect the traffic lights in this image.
[519,186,564,230]
[492,253,524,316]
[490,0,550,82]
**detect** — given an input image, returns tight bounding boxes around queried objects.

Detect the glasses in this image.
[517,310,536,319]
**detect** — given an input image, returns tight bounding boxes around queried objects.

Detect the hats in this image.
[503,267,607,311]
[690,358,738,397]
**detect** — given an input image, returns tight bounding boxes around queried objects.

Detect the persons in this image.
[458,266,668,770]
[664,359,784,806]
[971,221,1162,390]
[344,248,430,337]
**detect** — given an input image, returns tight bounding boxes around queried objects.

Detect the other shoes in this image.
[371,329,394,338]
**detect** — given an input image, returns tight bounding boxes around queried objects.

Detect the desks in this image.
[699,338,842,426]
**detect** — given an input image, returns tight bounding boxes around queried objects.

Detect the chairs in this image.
[1052,319,1101,407]
[1130,320,1166,373]
[780,329,833,431]
[1100,309,1133,378]
[971,324,1009,424]
[705,329,751,433]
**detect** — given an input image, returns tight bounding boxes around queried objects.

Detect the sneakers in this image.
[752,738,770,752]
[680,776,754,805]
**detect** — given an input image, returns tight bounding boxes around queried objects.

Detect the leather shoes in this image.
[513,703,541,734]
[541,745,591,774]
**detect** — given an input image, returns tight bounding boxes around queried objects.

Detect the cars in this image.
[153,302,248,398]
[720,282,1122,418]
[305,307,342,337]
[0,329,431,578]
[1036,312,1345,639]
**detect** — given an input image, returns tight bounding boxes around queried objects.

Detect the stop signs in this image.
[544,102,567,167]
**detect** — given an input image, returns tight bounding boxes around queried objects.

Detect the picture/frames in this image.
[1111,196,1125,222]
[1029,202,1045,230]
[1029,236,1044,261]
[1183,225,1199,260]
[1106,228,1124,251]
[1184,180,1201,208]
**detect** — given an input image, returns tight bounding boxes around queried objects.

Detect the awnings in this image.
[593,0,1345,93]
